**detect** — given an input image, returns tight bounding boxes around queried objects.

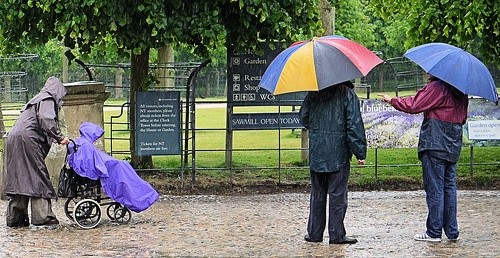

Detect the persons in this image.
[376,71,469,242]
[299,80,367,244]
[67,121,158,213]
[5,75,70,228]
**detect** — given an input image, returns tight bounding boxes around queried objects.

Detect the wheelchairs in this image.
[64,139,132,230]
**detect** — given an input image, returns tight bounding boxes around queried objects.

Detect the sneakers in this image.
[447,237,460,241]
[414,233,441,242]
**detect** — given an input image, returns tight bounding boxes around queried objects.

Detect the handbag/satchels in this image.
[57,168,77,197]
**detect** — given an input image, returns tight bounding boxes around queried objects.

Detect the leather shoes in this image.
[43,217,59,225]
[335,236,357,244]
[20,218,29,227]
[305,234,314,242]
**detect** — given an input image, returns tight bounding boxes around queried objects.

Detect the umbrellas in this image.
[258,35,384,95]
[402,42,499,106]
